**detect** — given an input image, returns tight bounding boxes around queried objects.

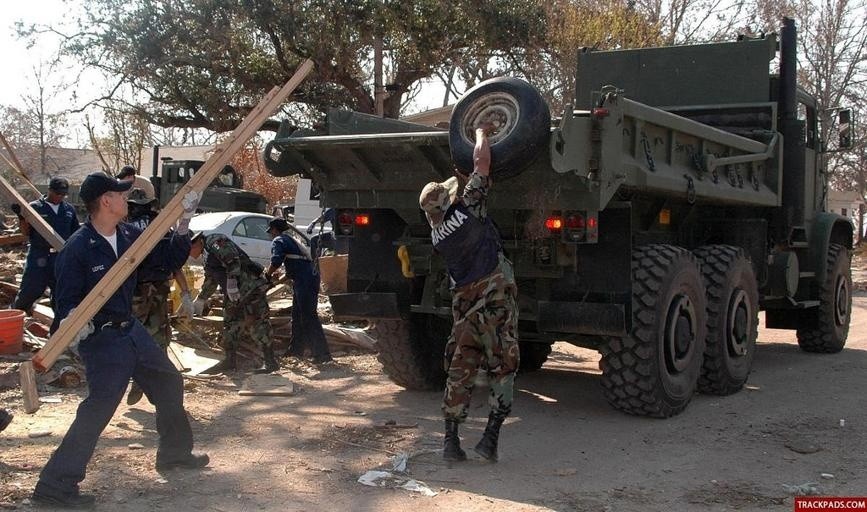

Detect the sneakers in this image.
[282,353,332,364]
[156,451,209,468]
[0,409,13,434]
[33,482,95,508]
[128,379,144,405]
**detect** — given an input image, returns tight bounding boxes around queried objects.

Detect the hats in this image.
[126,188,159,207]
[419,176,459,228]
[187,229,203,241]
[115,166,135,179]
[78,172,132,201]
[49,177,69,194]
[265,218,288,233]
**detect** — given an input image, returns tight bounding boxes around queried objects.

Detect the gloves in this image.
[11,204,24,220]
[177,191,203,236]
[265,272,271,283]
[60,308,95,347]
[227,278,240,302]
[179,289,205,322]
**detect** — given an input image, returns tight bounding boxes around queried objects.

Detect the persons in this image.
[117,166,156,214]
[10,177,80,312]
[265,217,331,364]
[419,123,520,462]
[188,230,279,373]
[126,186,172,405]
[33,173,209,507]
[306,193,335,235]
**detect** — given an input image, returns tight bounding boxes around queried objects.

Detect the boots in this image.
[474,416,502,462]
[213,353,236,370]
[443,420,466,461]
[253,349,279,373]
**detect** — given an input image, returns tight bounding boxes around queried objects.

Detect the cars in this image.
[182,211,313,268]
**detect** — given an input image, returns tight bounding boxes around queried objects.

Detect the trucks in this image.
[262,15,853,418]
[14,146,265,213]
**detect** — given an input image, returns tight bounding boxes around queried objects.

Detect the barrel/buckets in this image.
[0,309,26,353]
